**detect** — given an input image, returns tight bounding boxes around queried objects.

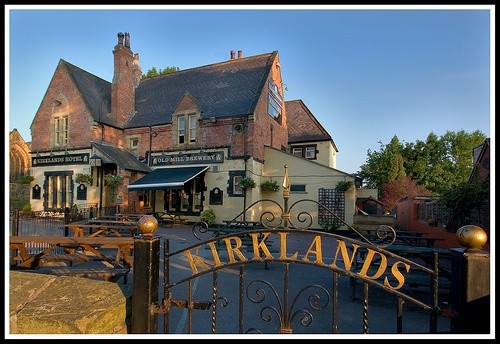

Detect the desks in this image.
[205,220,274,269]
[346,229,451,301]
[9,214,189,284]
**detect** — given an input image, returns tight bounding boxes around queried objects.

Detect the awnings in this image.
[126,164,211,191]
[90,140,153,173]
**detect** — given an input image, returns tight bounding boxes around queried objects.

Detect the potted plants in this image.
[260,180,280,192]
[336,180,354,191]
[104,174,123,187]
[76,174,93,184]
[200,209,216,227]
[239,177,256,188]
